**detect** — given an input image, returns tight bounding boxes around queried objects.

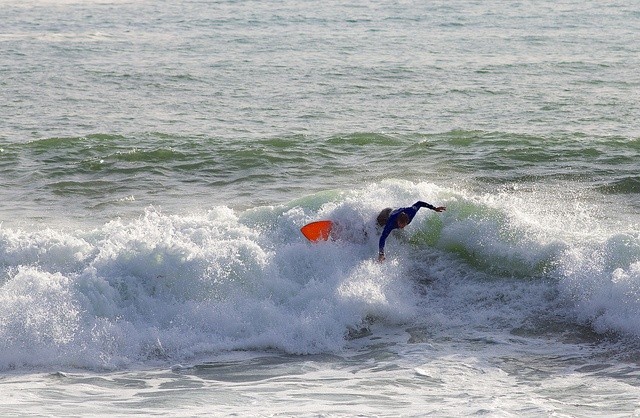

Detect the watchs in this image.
[379,252,384,255]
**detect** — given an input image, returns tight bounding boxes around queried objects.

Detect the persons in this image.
[377,201,446,263]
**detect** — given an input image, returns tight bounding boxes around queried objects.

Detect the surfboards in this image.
[300,219,334,245]
